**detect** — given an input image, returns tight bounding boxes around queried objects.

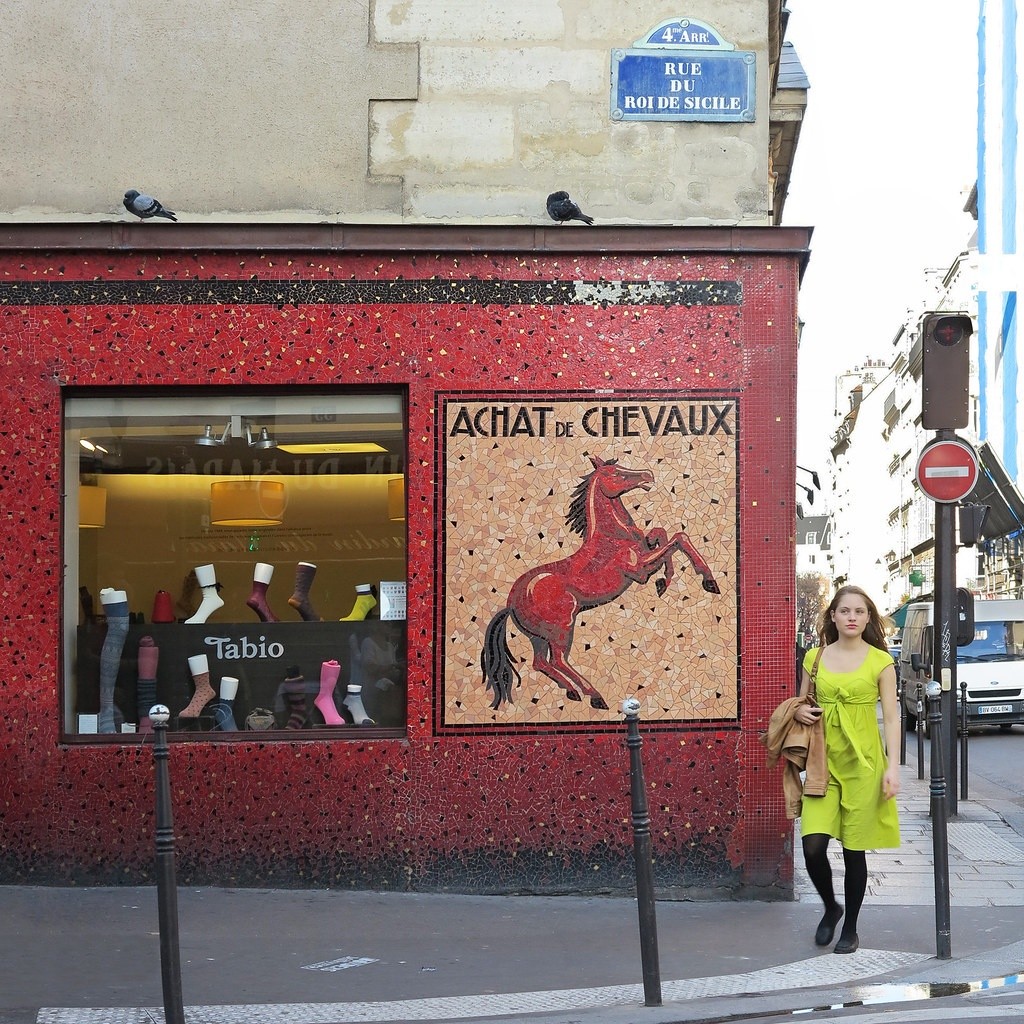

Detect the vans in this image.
[899,599,1024,739]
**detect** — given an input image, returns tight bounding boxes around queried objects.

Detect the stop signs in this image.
[915,441,980,504]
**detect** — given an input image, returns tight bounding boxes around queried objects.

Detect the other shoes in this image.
[834,932,859,954]
[815,902,843,945]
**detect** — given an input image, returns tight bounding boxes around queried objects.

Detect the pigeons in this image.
[546,192,597,224]
[123,191,177,223]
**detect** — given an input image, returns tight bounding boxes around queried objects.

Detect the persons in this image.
[342,684,375,725]
[246,562,281,622]
[793,585,900,954]
[214,676,240,732]
[183,563,224,624]
[175,574,198,616]
[179,655,216,718]
[97,589,129,735]
[288,560,325,622]
[339,584,377,621]
[313,660,345,725]
[281,664,312,729]
[137,638,159,734]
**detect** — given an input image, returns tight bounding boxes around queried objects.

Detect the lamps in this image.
[79,485,107,529]
[193,421,231,448]
[244,422,278,449]
[210,480,285,527]
[796,466,821,520]
[387,478,406,521]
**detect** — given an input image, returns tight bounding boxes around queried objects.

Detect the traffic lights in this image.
[960,505,991,545]
[923,316,973,430]
